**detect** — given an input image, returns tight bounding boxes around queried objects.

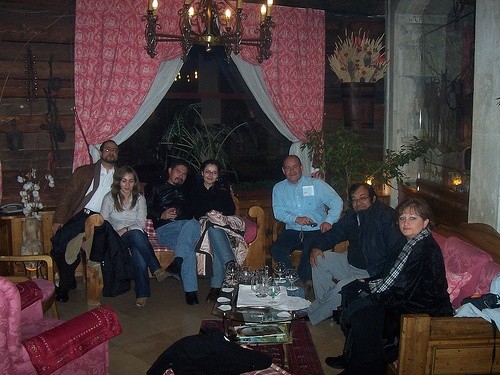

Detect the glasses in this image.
[103,148,119,153]
[350,194,371,203]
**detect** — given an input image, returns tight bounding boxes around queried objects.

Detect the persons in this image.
[238,325,283,337]
[147,159,201,305]
[90,165,171,307]
[49,140,122,302]
[327,197,452,375]
[186,159,240,304]
[301,183,405,326]
[267,154,343,289]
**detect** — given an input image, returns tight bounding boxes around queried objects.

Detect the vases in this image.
[20,218,43,271]
[342,83,376,128]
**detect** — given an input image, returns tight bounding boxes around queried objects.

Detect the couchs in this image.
[0,276,122,375]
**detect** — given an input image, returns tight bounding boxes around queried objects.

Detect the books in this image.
[234,324,287,342]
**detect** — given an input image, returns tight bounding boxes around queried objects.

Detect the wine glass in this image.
[226,261,300,291]
[169,207,177,222]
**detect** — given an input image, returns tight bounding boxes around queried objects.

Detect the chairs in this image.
[0,255,59,321]
[272,222,302,277]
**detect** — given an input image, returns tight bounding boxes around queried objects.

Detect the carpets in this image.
[199,320,325,375]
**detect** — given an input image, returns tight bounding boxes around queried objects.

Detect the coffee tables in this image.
[212,273,307,370]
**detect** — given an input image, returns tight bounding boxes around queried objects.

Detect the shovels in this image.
[6,119,23,151]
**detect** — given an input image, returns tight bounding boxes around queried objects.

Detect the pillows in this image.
[446,269,472,303]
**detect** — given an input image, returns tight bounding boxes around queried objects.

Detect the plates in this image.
[272,296,312,311]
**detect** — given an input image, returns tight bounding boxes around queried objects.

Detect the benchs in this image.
[334,223,500,375]
[86,184,265,305]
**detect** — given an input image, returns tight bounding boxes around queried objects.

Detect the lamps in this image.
[141,0,277,63]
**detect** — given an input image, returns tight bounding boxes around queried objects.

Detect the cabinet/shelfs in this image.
[0,207,56,277]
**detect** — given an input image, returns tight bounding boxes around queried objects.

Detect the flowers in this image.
[327,26,392,83]
[17,168,55,220]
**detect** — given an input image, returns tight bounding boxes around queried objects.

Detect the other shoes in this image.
[70,271,76,290]
[155,270,168,282]
[56,288,68,302]
[136,297,147,307]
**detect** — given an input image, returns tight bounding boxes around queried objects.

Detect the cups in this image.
[251,272,268,298]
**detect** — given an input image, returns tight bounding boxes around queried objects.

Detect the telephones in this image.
[1,203,23,214]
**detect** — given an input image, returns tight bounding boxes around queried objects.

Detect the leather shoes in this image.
[206,287,220,303]
[227,261,237,271]
[325,355,345,369]
[166,259,183,278]
[186,292,198,305]
